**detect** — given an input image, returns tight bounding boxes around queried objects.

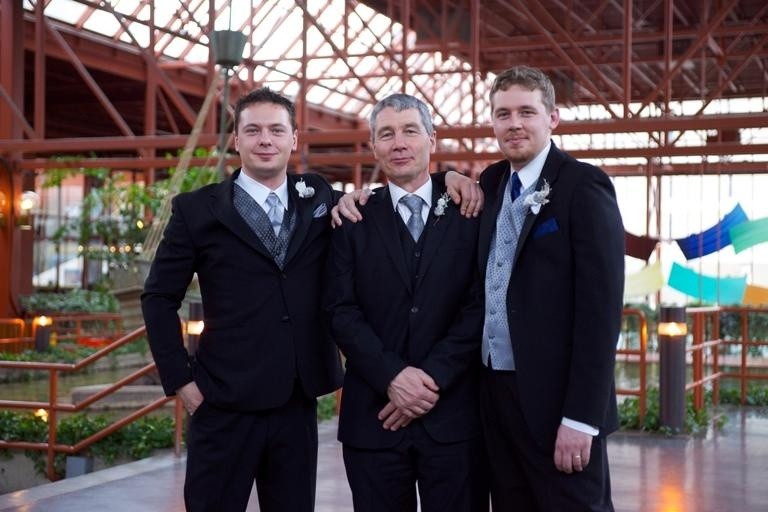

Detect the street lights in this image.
[211,30,247,186]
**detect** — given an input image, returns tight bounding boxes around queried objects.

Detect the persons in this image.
[329,63,621,511]
[328,94,491,511]
[140,86,485,512]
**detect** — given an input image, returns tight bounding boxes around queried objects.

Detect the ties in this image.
[266,193,283,234]
[510,170,523,202]
[398,195,425,240]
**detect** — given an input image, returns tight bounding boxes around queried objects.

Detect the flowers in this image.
[521,176,555,215]
[295,175,316,198]
[431,191,453,226]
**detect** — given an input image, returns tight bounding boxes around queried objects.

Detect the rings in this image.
[574,455,581,458]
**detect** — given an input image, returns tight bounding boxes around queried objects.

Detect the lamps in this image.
[13,189,44,232]
[34,309,53,353]
[658,305,688,427]
[187,300,204,356]
